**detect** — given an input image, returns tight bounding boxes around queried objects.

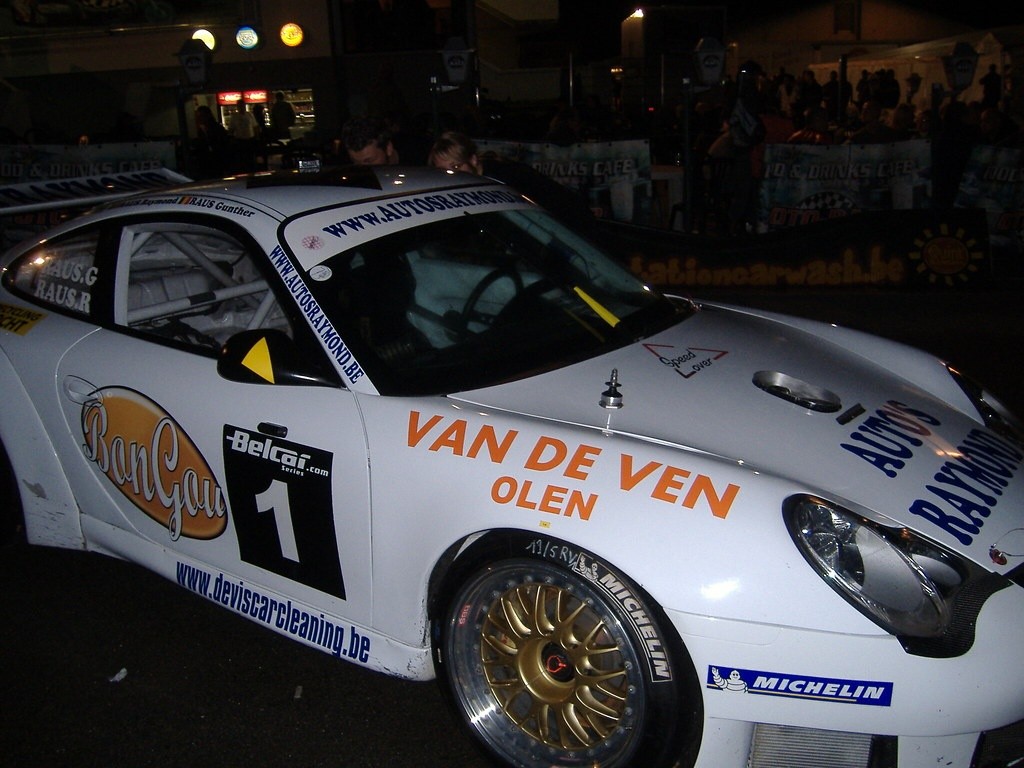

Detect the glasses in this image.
[450,161,466,171]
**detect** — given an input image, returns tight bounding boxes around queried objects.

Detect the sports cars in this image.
[0,165,1024,768]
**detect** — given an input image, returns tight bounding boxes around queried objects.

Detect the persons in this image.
[333,58,1024,244]
[106,113,148,142]
[229,100,260,157]
[195,105,230,178]
[271,93,296,132]
